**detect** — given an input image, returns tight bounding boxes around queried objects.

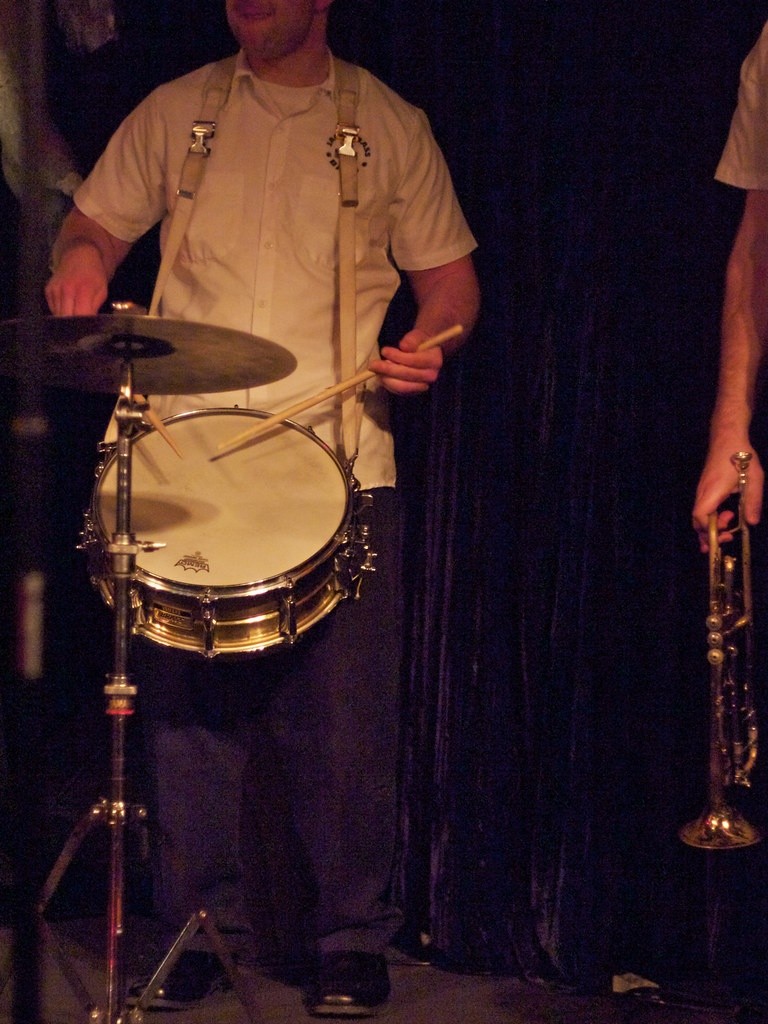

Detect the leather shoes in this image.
[305,950,393,1016]
[125,949,219,1009]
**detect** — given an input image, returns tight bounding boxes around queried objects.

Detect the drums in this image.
[71,406,377,662]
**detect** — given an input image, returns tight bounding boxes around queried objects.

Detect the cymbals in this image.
[0,312,300,399]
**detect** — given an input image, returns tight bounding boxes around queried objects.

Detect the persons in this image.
[680,20,768,556]
[46,0,480,1020]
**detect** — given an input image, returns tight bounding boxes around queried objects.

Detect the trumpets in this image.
[678,446,763,850]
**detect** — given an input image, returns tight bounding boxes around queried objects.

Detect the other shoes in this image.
[620,978,756,1023]
